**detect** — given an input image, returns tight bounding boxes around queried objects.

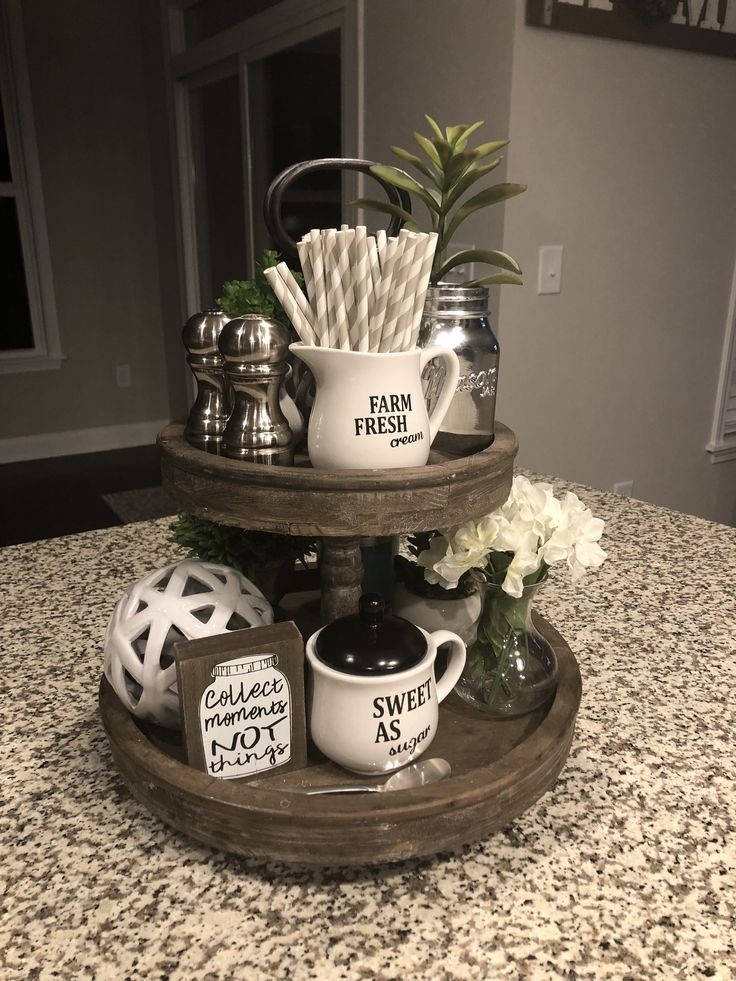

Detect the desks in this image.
[0,466,736,980]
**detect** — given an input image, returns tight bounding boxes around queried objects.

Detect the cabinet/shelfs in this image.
[99,415,582,866]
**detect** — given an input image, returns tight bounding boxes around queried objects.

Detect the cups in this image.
[305,591,468,778]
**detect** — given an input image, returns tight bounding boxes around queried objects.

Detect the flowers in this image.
[415,475,609,704]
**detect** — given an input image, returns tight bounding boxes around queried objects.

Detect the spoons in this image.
[279,757,451,795]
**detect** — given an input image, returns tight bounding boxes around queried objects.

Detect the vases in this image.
[445,571,558,718]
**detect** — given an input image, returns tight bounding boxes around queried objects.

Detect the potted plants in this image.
[393,529,482,647]
[165,511,317,606]
[214,248,311,443]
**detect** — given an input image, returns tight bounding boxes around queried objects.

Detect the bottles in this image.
[180,310,236,456]
[215,315,295,468]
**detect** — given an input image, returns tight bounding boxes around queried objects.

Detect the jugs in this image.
[288,341,460,470]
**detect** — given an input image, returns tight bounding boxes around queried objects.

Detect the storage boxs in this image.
[172,621,307,784]
